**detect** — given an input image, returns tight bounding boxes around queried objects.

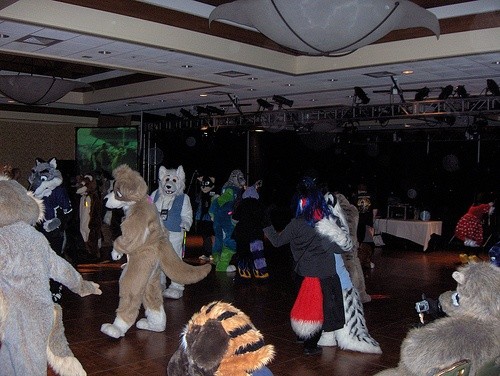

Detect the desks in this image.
[373,218,442,251]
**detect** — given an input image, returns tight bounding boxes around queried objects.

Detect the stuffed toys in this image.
[373,201,500,376]
[259,175,345,355]
[314,191,383,354]
[100,165,211,338]
[0,158,278,376]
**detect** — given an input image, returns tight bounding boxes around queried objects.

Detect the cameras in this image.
[415,300,430,312]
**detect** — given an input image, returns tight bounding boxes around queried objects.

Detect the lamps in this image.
[165,112,184,122]
[272,95,294,108]
[487,79,500,95]
[353,86,371,104]
[454,85,470,97]
[180,108,192,117]
[415,86,430,101]
[193,105,225,116]
[257,98,274,111]
[439,85,454,100]
